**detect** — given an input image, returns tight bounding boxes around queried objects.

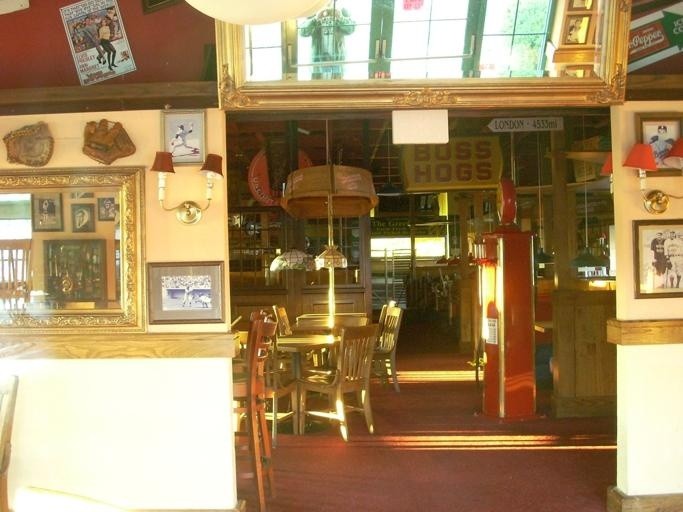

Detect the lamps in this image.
[572,115,597,267]
[270,221,315,273]
[391,109,450,144]
[534,130,555,269]
[149,151,223,224]
[623,138,682,214]
[282,165,379,221]
[314,194,347,269]
[595,236,609,266]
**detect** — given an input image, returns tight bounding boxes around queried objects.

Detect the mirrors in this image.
[214,0,632,108]
[1,165,147,331]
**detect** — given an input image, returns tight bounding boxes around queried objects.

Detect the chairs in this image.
[233,300,403,512]
[1,238,32,297]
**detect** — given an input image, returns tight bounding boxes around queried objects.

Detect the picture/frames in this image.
[31,192,119,299]
[143,262,226,323]
[634,112,683,176]
[159,111,206,165]
[632,219,683,298]
